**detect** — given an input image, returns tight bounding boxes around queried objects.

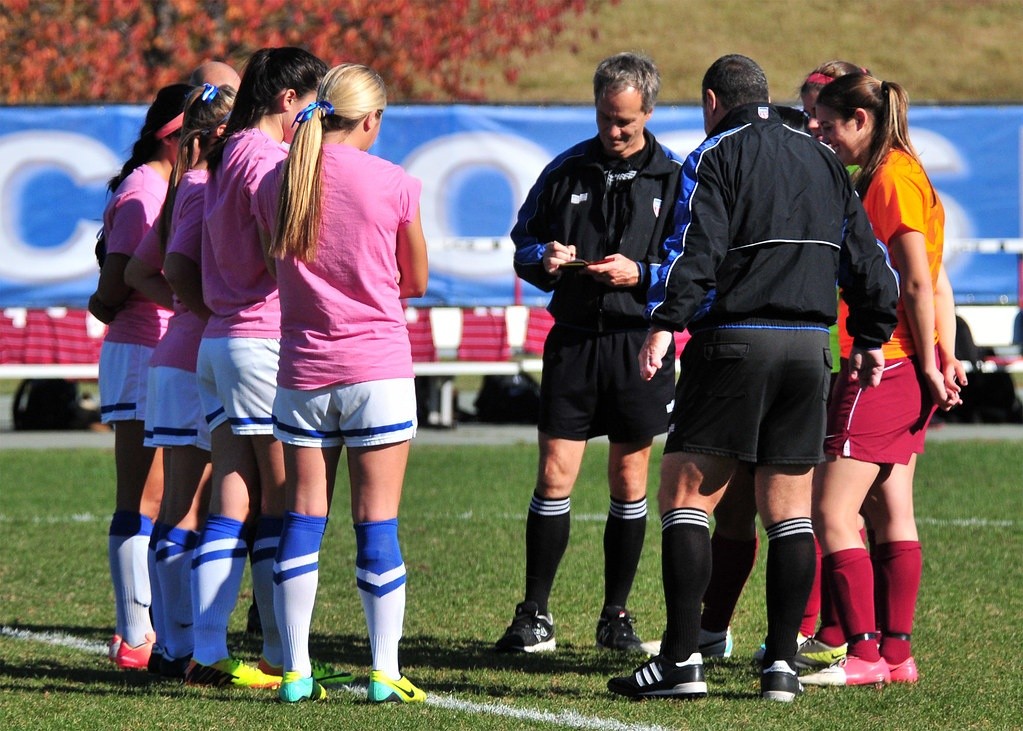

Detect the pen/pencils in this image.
[555,240,588,262]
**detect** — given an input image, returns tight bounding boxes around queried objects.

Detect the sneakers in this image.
[798,657,890,686]
[279,671,327,705]
[183,658,282,688]
[886,657,918,683]
[697,629,733,659]
[495,600,556,652]
[159,646,195,678]
[367,669,427,703]
[108,635,123,663]
[146,643,164,671]
[638,627,732,660]
[594,605,641,651]
[794,637,848,666]
[760,659,806,702]
[756,632,816,659]
[257,656,356,684]
[116,633,157,668]
[606,653,708,696]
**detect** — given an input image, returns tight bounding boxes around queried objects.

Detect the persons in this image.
[639,55,871,667]
[796,72,968,690]
[492,50,718,652]
[605,54,902,704]
[88,47,431,704]
